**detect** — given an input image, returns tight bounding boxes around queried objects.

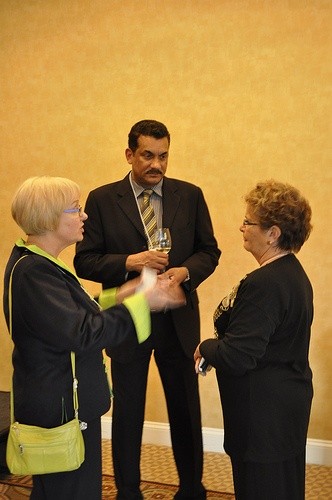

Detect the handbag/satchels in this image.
[7,418,84,476]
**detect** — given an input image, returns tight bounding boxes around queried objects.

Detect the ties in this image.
[141,190,159,248]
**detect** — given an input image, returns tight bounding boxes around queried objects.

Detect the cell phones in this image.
[198,357,212,371]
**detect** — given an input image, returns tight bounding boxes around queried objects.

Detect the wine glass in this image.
[153,227,171,254]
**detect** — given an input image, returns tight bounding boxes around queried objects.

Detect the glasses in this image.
[63,206,82,212]
[243,219,258,226]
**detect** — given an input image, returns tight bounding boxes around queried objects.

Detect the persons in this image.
[73,120,224,500]
[3,176,187,500]
[194,180,314,500]
